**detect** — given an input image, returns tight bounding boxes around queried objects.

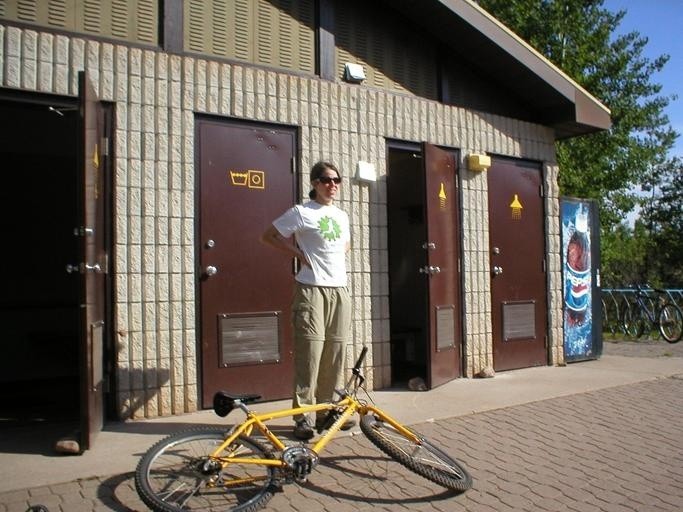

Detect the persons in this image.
[259,161,355,440]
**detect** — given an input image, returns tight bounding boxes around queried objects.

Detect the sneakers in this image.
[316,409,356,431]
[295,421,314,439]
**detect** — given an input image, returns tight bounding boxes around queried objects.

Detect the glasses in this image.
[314,177,341,183]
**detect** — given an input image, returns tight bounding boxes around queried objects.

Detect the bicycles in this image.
[601,278,683,344]
[134,346,472,511]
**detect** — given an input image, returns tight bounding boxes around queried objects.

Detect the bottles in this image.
[564,208,591,327]
